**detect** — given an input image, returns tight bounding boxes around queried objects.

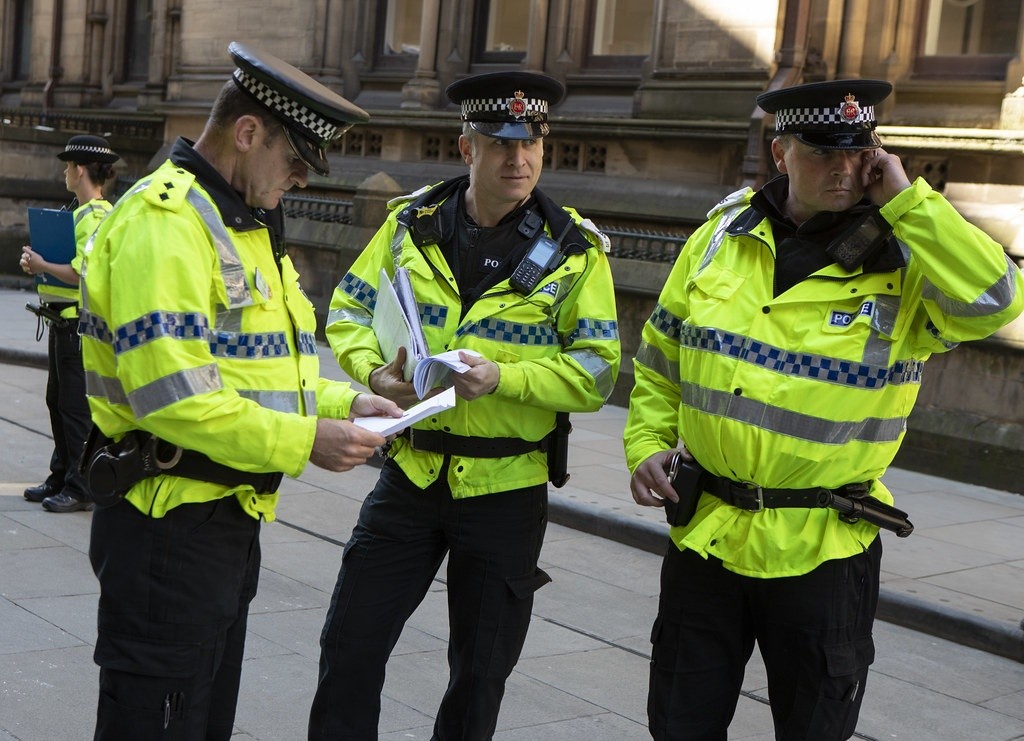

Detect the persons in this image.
[75,41,404,741]
[306,72,621,741]
[18,135,123,512]
[625,80,1024,741]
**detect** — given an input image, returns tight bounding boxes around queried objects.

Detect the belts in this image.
[696,462,875,513]
[403,424,554,459]
[136,430,284,493]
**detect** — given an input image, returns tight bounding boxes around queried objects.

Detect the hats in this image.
[757,78,895,150]
[57,135,121,164]
[227,41,371,177]
[446,70,568,141]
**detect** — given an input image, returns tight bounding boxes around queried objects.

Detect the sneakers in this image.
[24,482,58,502]
[43,490,95,512]
